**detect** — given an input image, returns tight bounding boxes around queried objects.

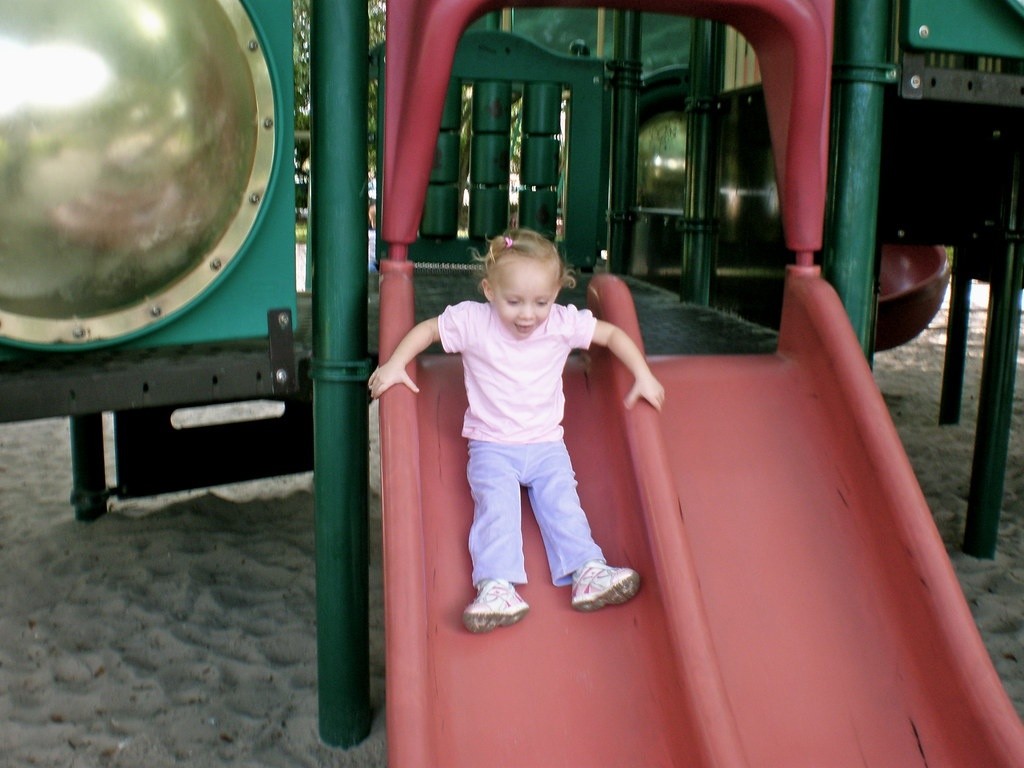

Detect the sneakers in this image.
[462,580,530,633]
[571,560,641,612]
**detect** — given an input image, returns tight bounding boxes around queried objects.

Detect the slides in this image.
[874,242,951,353]
[383,283,1024,768]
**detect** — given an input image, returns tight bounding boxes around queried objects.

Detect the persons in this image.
[367,226,665,633]
